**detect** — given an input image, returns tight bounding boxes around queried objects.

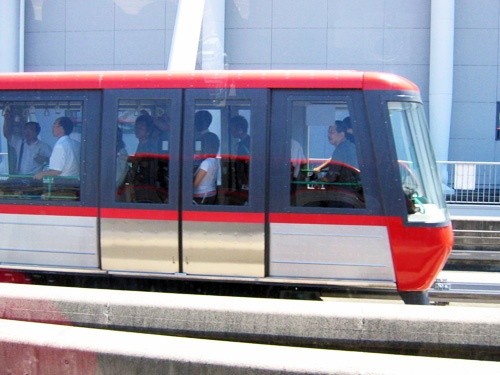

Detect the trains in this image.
[1,72,457,307]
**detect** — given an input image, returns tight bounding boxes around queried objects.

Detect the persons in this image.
[3,111,52,187]
[116,111,359,207]
[33,117,82,194]
